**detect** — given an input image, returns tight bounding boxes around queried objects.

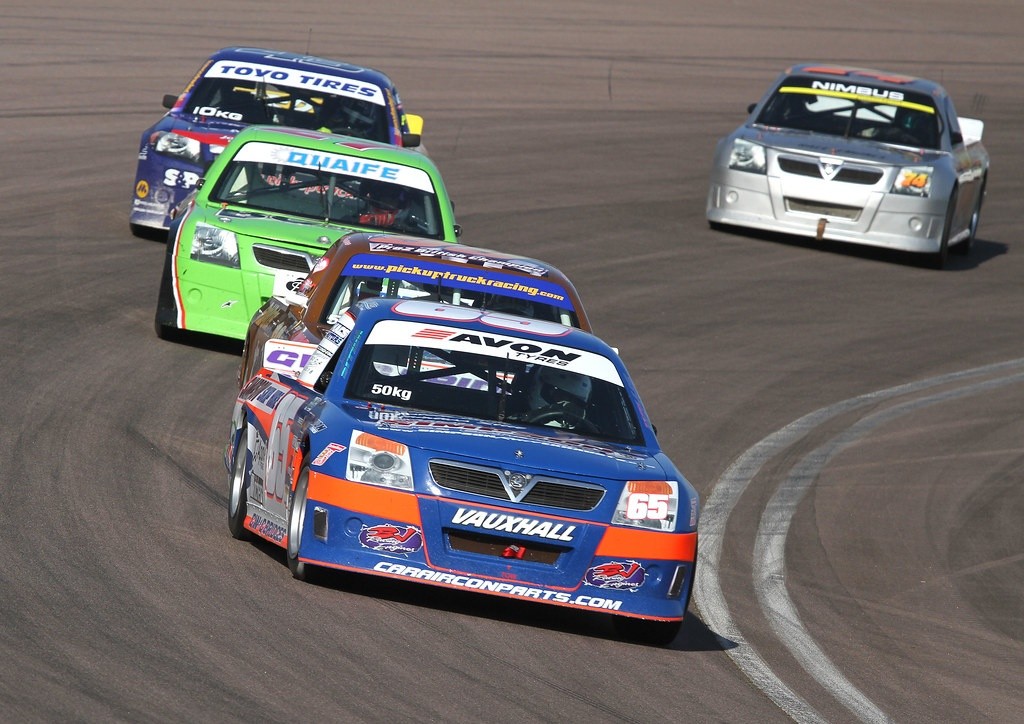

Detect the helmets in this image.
[488,293,535,319]
[528,365,591,430]
[357,179,412,227]
[331,94,381,138]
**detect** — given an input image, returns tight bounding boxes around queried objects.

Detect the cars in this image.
[153,124,464,346]
[129,45,424,241]
[705,61,992,261]
[235,230,595,399]
[222,297,700,646]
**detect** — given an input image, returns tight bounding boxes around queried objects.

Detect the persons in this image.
[338,180,428,238]
[322,96,379,140]
[504,364,591,434]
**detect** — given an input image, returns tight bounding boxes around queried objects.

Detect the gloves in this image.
[358,213,394,227]
